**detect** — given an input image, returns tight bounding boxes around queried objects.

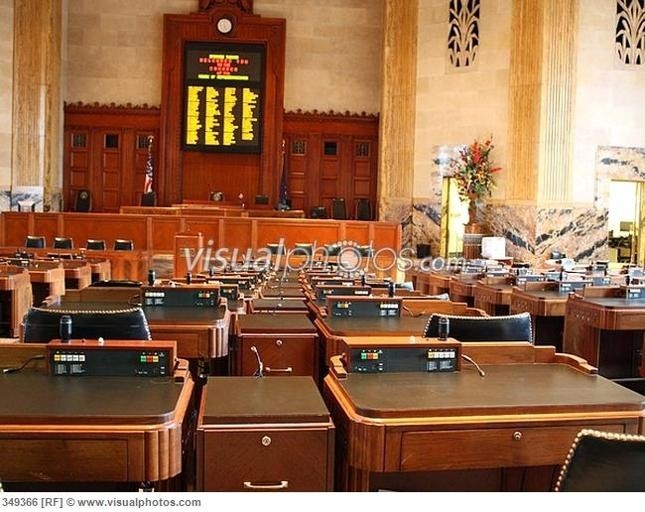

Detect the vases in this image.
[460,219,483,258]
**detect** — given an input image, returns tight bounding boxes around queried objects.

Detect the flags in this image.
[278,144,291,210]
[142,146,154,196]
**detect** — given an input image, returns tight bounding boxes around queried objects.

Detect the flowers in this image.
[448,135,505,227]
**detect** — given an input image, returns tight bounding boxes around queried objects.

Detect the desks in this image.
[0,237,643,491]
